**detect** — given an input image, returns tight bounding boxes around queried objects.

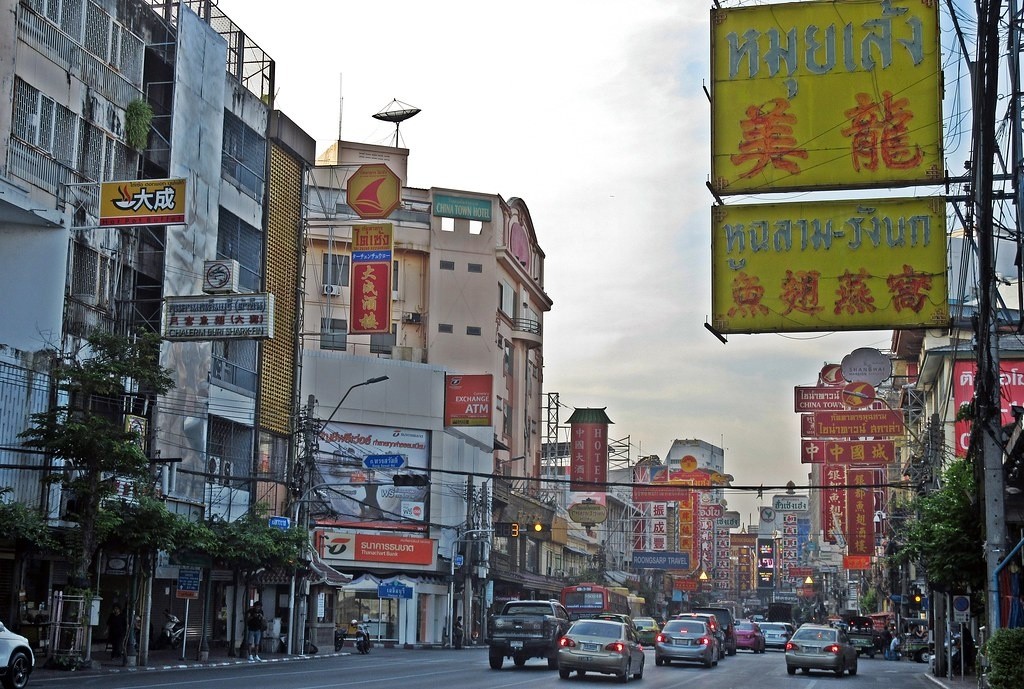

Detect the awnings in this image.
[994,537,1024,575]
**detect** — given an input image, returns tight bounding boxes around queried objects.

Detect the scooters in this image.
[159,609,185,649]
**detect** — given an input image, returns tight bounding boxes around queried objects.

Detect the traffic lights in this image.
[914,589,922,609]
[494,522,519,537]
[393,474,429,486]
[526,523,551,532]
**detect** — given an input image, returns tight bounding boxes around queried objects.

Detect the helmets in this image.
[351,619,358,627]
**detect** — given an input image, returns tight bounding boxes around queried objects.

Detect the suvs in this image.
[690,607,740,656]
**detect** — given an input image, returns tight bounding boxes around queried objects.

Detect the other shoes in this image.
[254,654,261,660]
[247,655,254,660]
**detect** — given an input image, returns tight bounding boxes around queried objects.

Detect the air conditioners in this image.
[322,284,337,295]
[219,459,233,486]
[62,459,77,486]
[203,454,220,483]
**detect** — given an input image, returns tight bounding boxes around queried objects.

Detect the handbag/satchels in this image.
[259,611,268,628]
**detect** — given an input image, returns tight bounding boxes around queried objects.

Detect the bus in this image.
[561,582,631,618]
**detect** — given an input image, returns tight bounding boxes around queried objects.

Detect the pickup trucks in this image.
[844,616,879,658]
[489,599,580,671]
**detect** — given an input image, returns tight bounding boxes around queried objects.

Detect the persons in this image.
[838,625,849,644]
[107,602,126,660]
[246,600,264,660]
[454,616,463,650]
[880,624,927,656]
[829,620,834,626]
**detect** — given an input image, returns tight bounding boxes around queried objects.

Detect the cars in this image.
[0,620,35,689]
[555,613,729,683]
[785,622,858,678]
[734,620,798,654]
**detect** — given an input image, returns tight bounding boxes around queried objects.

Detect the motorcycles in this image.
[350,618,373,655]
[897,617,929,663]
[335,622,348,652]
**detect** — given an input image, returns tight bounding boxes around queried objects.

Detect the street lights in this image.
[475,456,526,510]
[292,373,390,654]
[842,390,946,676]
